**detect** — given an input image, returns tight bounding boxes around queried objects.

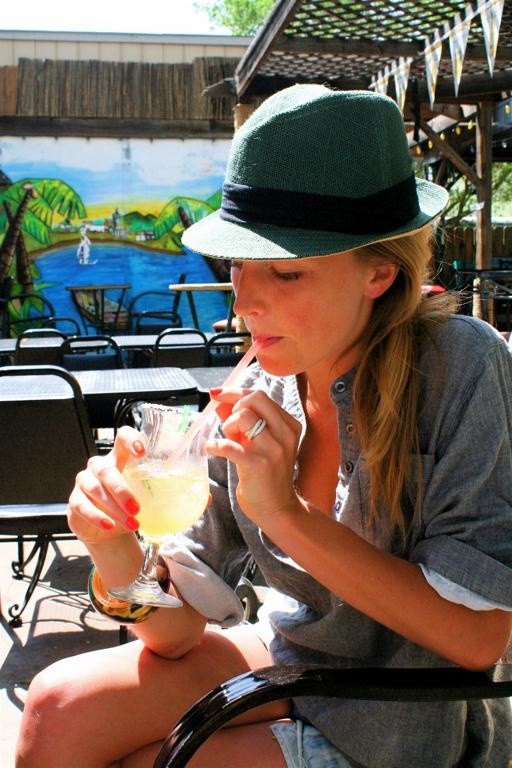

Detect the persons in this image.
[15,81,512,768]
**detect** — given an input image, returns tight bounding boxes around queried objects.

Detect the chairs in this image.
[152,664,512,768]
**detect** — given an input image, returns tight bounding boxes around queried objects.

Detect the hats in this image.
[178,79,452,263]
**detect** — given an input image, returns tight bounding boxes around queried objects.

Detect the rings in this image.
[244,418,267,441]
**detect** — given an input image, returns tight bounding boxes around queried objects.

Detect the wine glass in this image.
[106,402,220,612]
